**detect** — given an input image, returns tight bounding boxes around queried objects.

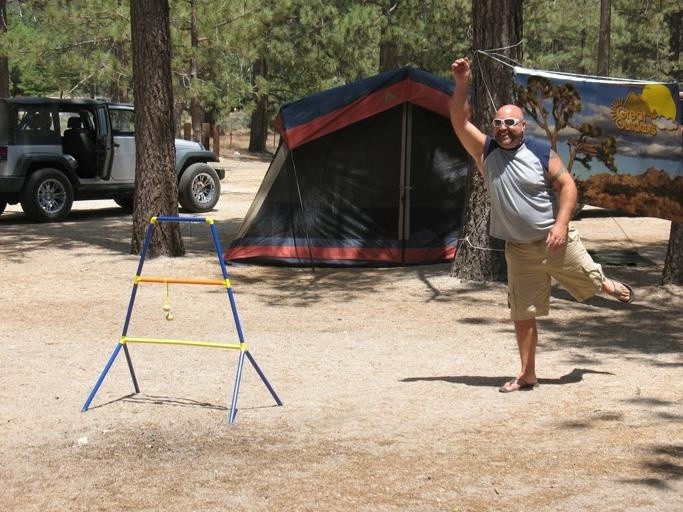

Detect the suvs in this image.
[0,97,225,223]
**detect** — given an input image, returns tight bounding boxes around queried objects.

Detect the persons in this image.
[450,57,636,393]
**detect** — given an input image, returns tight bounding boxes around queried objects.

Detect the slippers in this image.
[499,375,539,393]
[610,279,636,304]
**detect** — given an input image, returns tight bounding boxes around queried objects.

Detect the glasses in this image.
[491,118,521,126]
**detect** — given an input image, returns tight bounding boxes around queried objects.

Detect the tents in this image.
[224,66,473,268]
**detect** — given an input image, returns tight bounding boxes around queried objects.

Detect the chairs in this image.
[24,113,52,135]
[66,116,82,129]
